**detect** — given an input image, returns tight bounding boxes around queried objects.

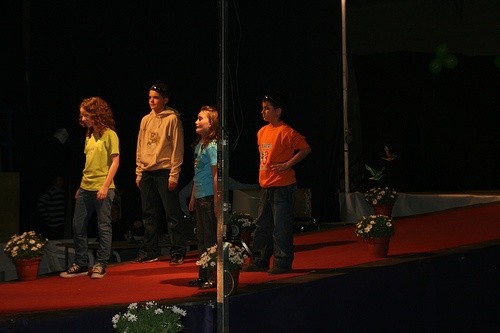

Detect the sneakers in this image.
[168,254,184,264]
[59,263,89,278]
[129,256,159,264]
[91,264,106,278]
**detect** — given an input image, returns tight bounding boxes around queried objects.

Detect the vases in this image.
[240,232,250,245]
[374,203,392,218]
[211,269,240,294]
[15,256,42,281]
[364,237,390,257]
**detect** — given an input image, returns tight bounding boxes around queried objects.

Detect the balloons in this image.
[495,57,500,67]
[429,58,443,74]
[435,44,449,59]
[442,55,457,69]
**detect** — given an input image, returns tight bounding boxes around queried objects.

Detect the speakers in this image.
[231,188,312,222]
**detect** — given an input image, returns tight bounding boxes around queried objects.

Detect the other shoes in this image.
[188,278,207,286]
[202,280,215,288]
[242,263,269,272]
[268,267,291,274]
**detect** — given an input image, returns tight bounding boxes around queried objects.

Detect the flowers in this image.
[196,242,249,271]
[227,209,258,232]
[364,187,400,206]
[112,301,187,333]
[353,214,395,240]
[1,230,49,261]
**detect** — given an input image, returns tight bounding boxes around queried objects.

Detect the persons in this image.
[189,106,218,287]
[60,96,120,278]
[136,84,186,264]
[242,94,311,275]
[35,174,65,235]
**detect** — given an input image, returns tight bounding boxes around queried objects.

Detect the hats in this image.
[150,83,168,97]
